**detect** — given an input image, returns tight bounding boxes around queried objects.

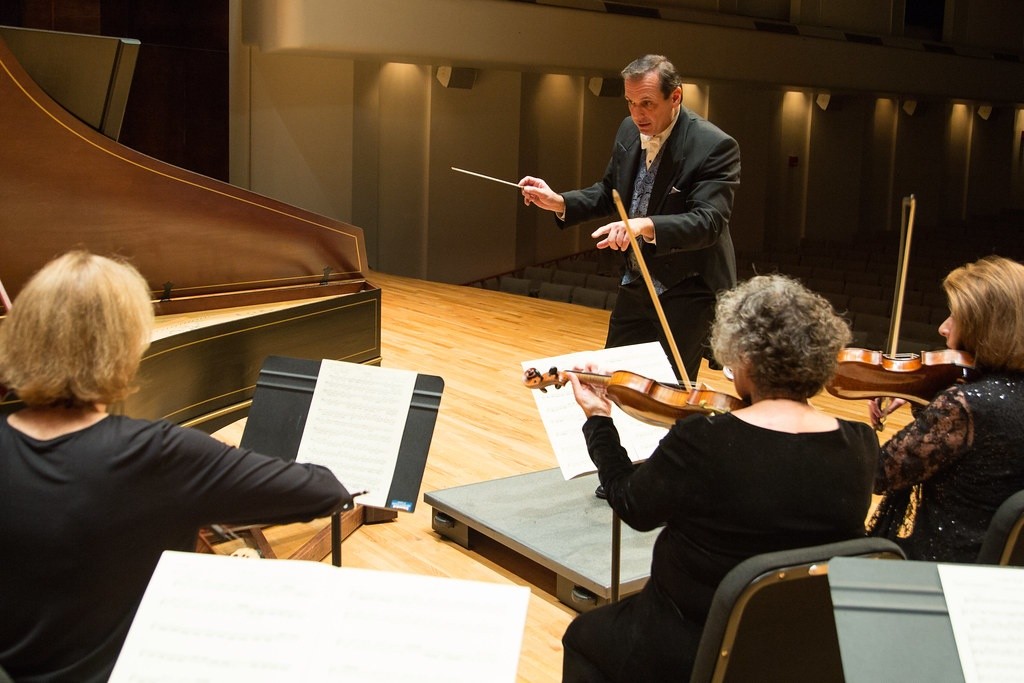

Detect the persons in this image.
[562,273,879,683]
[1,253,347,683]
[520,56,741,498]
[869,255,1024,564]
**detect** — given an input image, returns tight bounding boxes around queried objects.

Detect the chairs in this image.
[977,491,1024,567]
[480,204,1024,353]
[690,538,909,683]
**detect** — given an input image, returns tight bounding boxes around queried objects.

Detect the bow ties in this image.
[641,137,660,150]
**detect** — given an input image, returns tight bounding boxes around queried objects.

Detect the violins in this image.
[724,347,976,408]
[522,363,751,430]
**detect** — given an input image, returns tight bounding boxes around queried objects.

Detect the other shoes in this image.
[596,485,608,498]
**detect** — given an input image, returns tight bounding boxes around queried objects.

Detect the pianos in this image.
[0,24,387,438]
